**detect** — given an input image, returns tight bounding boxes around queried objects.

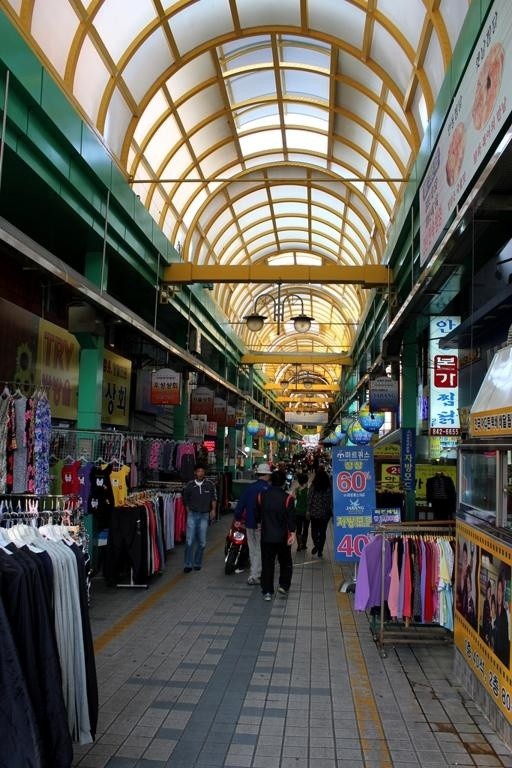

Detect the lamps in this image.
[280,363,314,389]
[242,280,315,333]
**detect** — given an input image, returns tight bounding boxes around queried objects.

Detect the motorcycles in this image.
[283,464,296,491]
[223,500,253,575]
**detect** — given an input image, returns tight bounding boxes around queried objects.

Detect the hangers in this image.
[111,488,183,511]
[1,493,82,517]
[0,509,92,579]
[0,380,51,409]
[361,527,456,558]
[49,450,131,472]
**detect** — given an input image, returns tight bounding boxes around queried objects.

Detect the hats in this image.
[254,464,273,474]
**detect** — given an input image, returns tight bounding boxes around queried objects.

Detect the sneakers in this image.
[297,544,323,556]
[183,566,201,573]
[247,575,289,601]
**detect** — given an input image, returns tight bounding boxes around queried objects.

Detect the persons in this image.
[232,463,273,586]
[425,471,456,520]
[455,543,509,670]
[180,464,218,573]
[237,447,333,557]
[254,470,298,601]
